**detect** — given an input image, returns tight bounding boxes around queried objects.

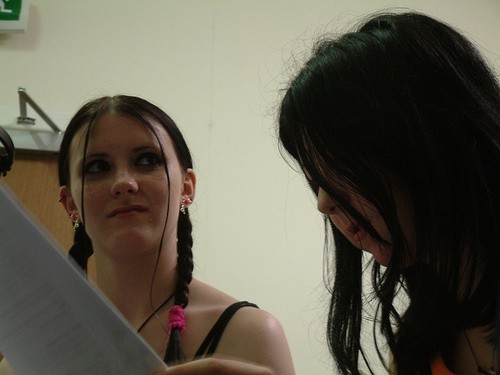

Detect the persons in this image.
[0,95,295,375]
[277,8,500,375]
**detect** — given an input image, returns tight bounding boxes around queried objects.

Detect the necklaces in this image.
[462,329,497,375]
[80,263,175,334]
